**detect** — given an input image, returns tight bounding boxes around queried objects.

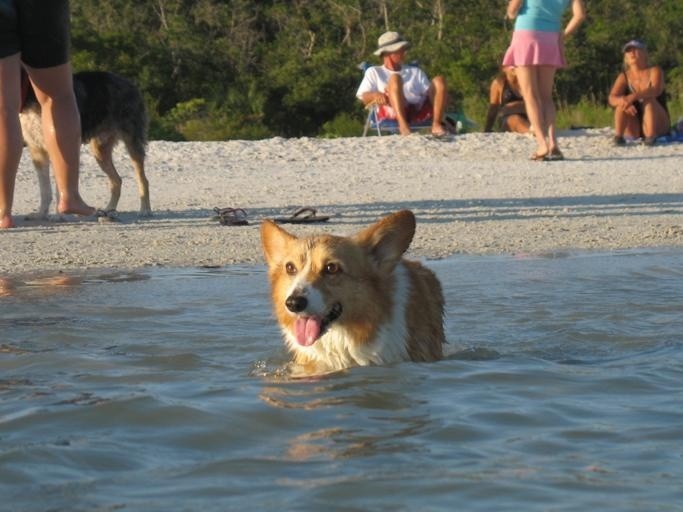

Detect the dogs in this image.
[259,209,450,378]
[19,64,154,218]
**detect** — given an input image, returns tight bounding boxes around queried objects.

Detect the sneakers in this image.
[640,136,657,146]
[614,136,626,146]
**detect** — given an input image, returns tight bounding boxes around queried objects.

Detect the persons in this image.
[0,0,94,231]
[504,1,585,159]
[608,41,671,148]
[356,31,450,138]
[482,59,535,136]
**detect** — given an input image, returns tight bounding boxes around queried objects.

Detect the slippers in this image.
[95,208,121,223]
[549,151,564,160]
[273,208,329,224]
[527,151,550,161]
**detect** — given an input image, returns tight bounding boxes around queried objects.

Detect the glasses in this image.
[213,206,249,226]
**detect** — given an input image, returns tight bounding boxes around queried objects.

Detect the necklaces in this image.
[635,74,642,84]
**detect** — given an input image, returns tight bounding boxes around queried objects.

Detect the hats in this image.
[623,39,645,52]
[370,29,412,57]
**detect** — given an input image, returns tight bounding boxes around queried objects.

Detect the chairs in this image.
[356,57,469,138]
